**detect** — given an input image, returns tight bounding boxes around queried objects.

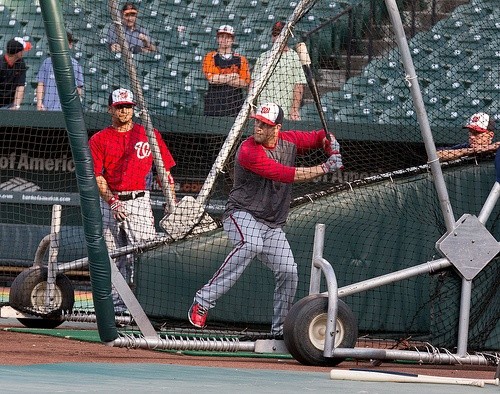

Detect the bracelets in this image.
[111,203,122,210]
[108,195,119,205]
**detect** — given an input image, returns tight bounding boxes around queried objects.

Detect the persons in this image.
[436,111,500,162]
[0,36,33,110]
[35,31,83,111]
[188,101,344,335]
[248,21,307,120]
[88,88,176,317]
[203,25,251,118]
[106,2,155,52]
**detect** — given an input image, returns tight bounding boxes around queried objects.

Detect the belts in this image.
[119,192,145,200]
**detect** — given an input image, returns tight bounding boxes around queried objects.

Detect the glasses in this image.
[115,105,135,110]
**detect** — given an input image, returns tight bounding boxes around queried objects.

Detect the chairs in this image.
[0,0,500,127]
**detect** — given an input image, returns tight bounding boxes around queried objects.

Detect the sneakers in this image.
[188,298,207,329]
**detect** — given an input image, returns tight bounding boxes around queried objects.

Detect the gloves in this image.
[321,154,344,173]
[323,136,340,154]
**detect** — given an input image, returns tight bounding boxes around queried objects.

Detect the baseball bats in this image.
[295,41,332,141]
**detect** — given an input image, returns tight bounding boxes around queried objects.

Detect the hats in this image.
[461,112,496,134]
[272,22,292,37]
[219,25,235,37]
[249,103,284,126]
[122,4,138,15]
[6,37,31,54]
[66,30,79,43]
[108,89,136,107]
